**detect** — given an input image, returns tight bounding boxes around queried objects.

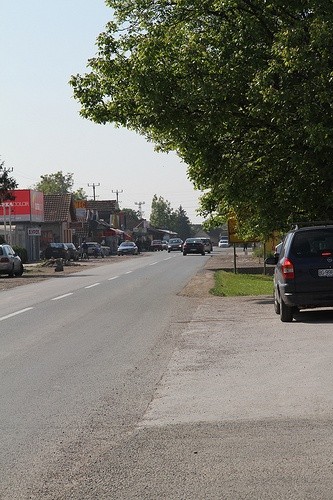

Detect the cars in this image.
[200,238,213,254]
[117,242,139,256]
[274,242,283,257]
[168,238,183,253]
[218,240,230,248]
[44,243,71,263]
[151,240,163,252]
[64,243,79,262]
[76,242,111,256]
[161,240,169,251]
[182,238,206,256]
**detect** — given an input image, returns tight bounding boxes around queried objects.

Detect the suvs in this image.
[0,244,24,278]
[264,224,333,322]
